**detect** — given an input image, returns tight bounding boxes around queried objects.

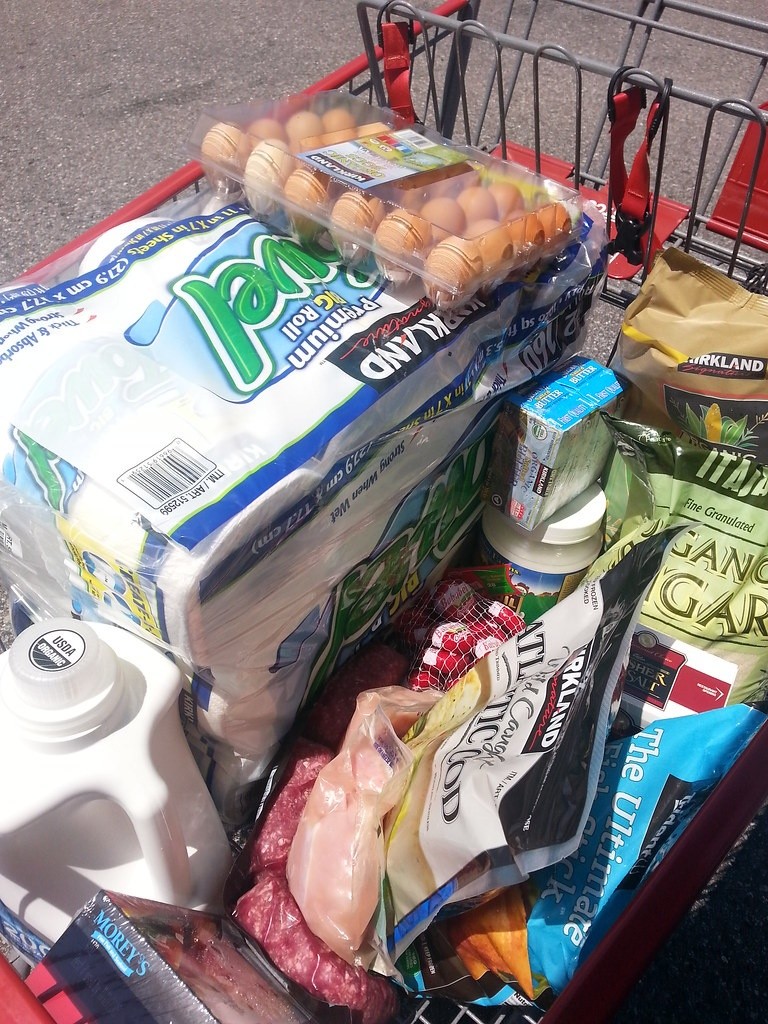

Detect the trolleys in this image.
[2,1,768,1024]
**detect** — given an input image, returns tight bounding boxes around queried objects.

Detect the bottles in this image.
[0,618,232,970]
[472,480,606,628]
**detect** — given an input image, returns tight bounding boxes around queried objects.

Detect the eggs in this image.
[201,106,572,307]
